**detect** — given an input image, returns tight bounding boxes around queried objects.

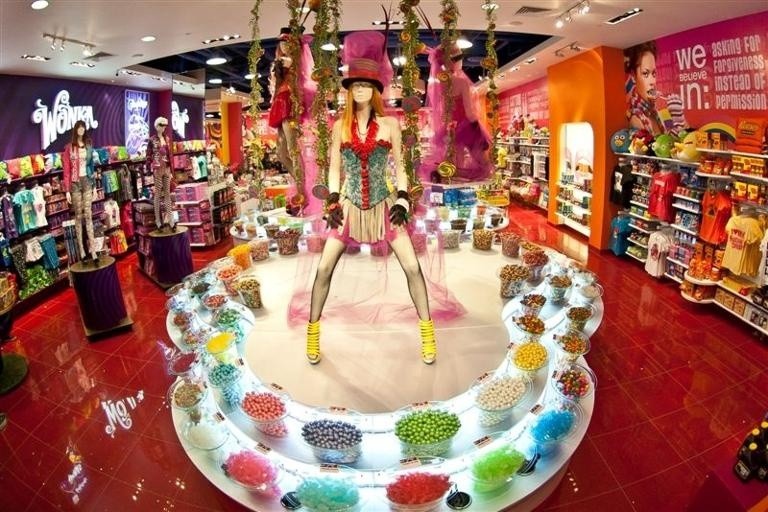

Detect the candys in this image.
[408,185,424,210]
[312,184,329,200]
[437,160,457,185]
[170,226,599,511]
[291,193,304,206]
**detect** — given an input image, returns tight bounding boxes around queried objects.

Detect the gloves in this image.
[665,94,689,137]
[387,190,411,225]
[323,192,345,226]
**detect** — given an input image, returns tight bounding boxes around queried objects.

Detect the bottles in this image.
[672,231,696,264]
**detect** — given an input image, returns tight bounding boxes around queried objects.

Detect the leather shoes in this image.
[92,254,100,264]
[78,254,88,265]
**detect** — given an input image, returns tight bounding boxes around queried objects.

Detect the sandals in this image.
[305,319,321,365]
[419,318,438,365]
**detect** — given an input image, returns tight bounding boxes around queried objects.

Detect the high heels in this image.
[168,222,176,233]
[154,221,163,233]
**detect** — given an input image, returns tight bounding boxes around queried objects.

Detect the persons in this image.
[269,26,306,202]
[302,55,441,366]
[434,41,489,177]
[145,117,180,234]
[623,40,696,137]
[61,120,102,268]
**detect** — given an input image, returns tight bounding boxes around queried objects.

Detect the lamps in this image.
[42,34,97,58]
[554,1,593,29]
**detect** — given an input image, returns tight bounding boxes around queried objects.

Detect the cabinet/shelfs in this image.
[614,146,768,340]
[163,240,606,511]
[174,173,236,247]
[0,149,210,319]
[412,128,595,240]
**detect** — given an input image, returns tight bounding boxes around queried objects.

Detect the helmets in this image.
[341,57,386,93]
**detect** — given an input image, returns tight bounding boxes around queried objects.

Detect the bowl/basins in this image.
[167,205,602,511]
